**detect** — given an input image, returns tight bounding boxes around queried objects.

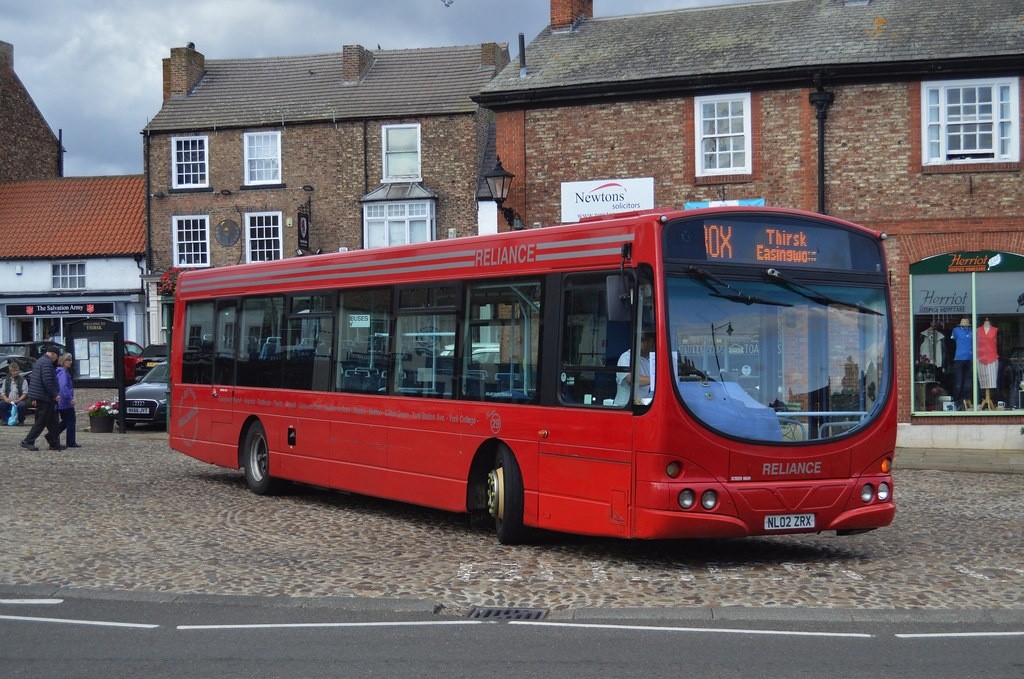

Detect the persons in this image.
[614,326,655,405]
[0,362,29,426]
[976,320,1000,389]
[43,353,82,448]
[949,318,973,399]
[916,327,946,373]
[19,347,67,451]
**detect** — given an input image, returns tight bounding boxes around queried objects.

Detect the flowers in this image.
[84,399,119,417]
[917,354,929,371]
[157,265,195,297]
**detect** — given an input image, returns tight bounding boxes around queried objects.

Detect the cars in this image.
[0,340,69,403]
[113,361,174,432]
[122,342,170,383]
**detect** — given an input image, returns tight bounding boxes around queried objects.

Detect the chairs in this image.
[204,334,520,402]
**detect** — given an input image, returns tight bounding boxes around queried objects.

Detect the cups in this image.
[998,401,1006,407]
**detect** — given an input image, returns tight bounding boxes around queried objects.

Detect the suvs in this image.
[439,341,499,366]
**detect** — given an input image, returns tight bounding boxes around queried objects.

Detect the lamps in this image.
[153,190,164,198]
[220,188,232,195]
[294,248,304,256]
[484,154,515,225]
[316,248,324,255]
[303,184,314,194]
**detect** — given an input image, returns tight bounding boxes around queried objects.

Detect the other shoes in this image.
[44,433,53,444]
[49,445,66,450]
[20,439,40,451]
[67,442,81,447]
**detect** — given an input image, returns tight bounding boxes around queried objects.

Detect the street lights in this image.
[711,320,733,391]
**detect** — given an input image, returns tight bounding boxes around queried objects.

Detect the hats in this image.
[47,346,60,354]
[956,318,970,326]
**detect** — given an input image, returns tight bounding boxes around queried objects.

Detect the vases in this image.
[161,296,177,303]
[916,370,924,381]
[89,416,115,432]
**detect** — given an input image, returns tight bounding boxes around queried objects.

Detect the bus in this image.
[171,205,898,544]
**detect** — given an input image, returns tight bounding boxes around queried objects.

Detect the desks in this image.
[914,380,940,411]
